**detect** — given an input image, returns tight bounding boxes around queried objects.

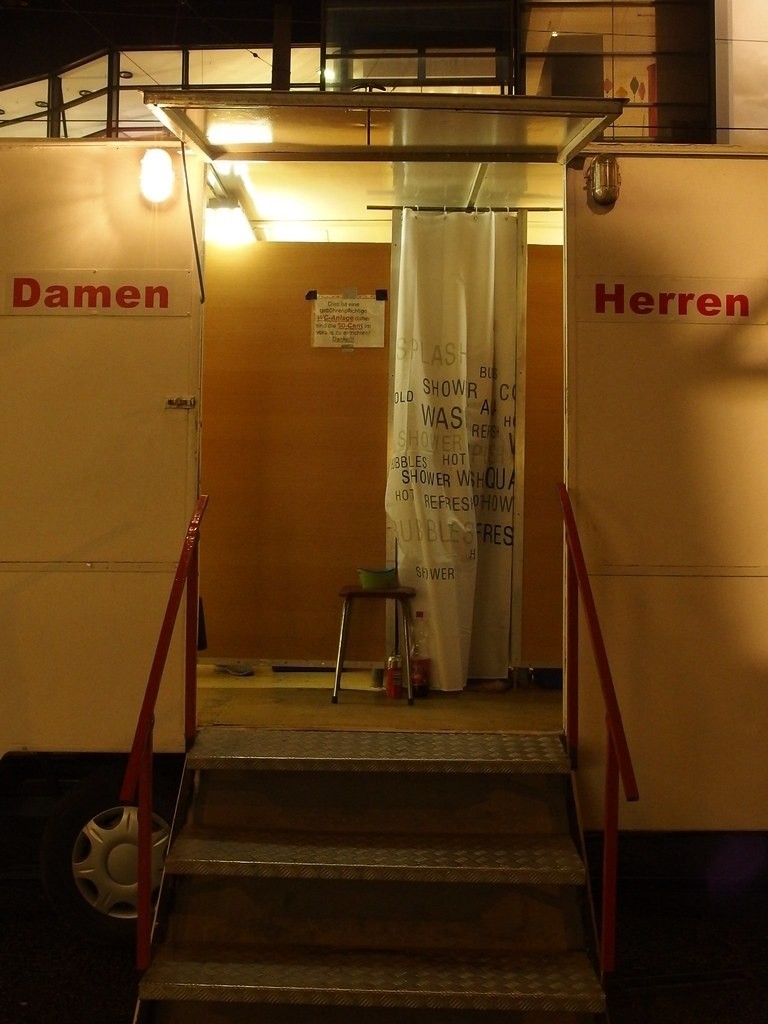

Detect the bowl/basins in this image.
[356,568,397,591]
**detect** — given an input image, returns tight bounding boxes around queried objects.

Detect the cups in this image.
[387,654,403,699]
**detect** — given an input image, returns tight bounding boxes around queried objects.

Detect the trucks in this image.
[2,80,768,1023]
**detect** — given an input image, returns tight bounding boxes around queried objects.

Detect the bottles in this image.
[410,611,430,699]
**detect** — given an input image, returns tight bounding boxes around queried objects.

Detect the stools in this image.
[331,584,417,705]
